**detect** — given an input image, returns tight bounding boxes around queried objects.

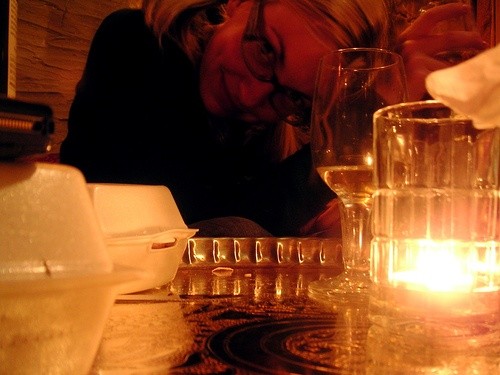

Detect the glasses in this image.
[241,0,338,131]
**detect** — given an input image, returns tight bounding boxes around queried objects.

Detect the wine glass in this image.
[307,48,409,295]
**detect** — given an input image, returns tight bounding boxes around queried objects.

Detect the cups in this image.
[417,0,473,57]
[371,100,474,290]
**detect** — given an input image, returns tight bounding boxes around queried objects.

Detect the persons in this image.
[58,0,488,237]
